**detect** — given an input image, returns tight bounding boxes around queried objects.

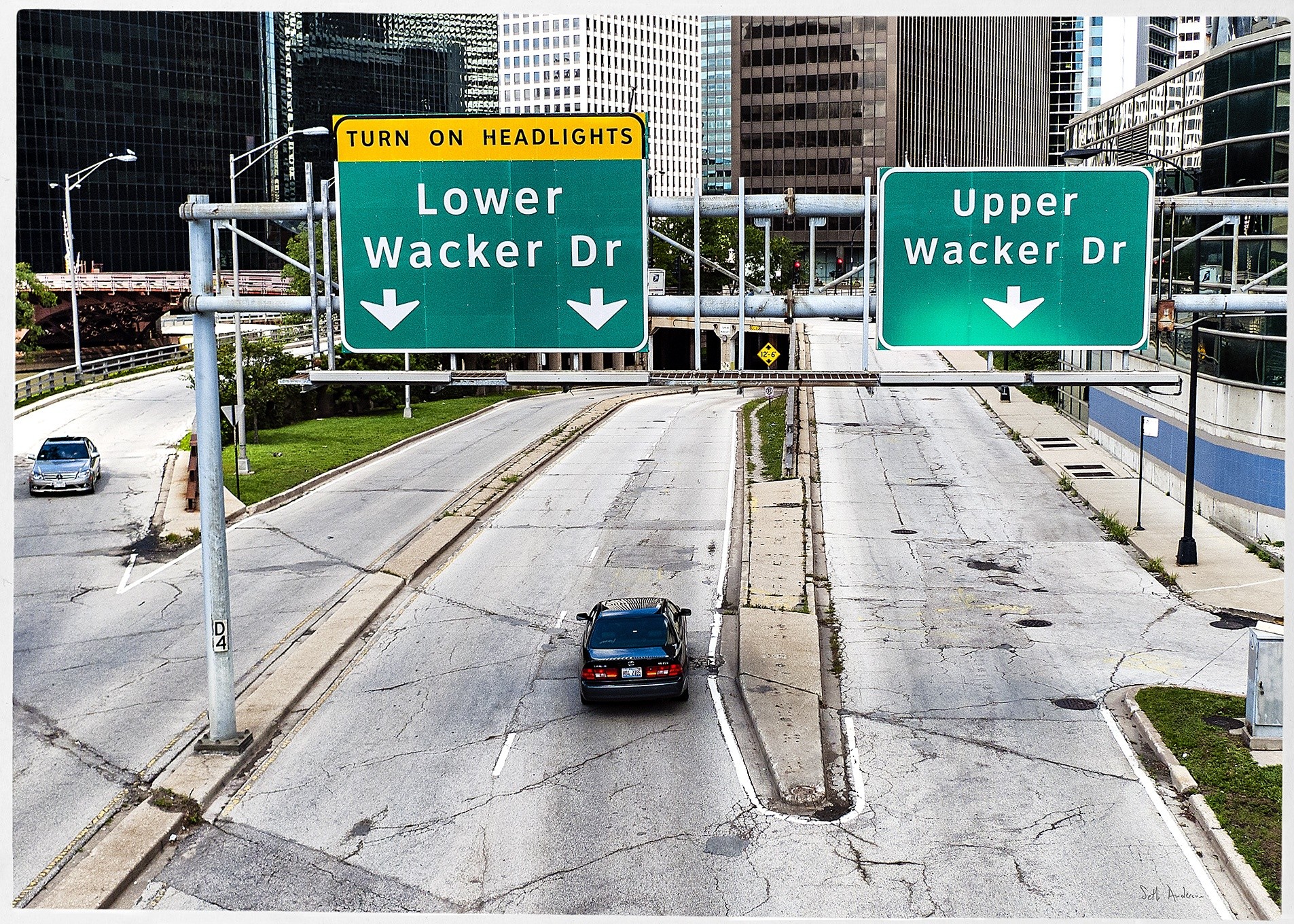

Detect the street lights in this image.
[1064,145,1200,568]
[230,125,330,476]
[64,153,137,369]
[851,221,871,297]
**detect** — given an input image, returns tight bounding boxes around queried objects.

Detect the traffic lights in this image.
[1153,259,1158,277]
[1162,256,1170,279]
[834,255,843,278]
[794,259,801,284]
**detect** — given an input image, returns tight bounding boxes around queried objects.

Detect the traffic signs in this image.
[330,106,653,354]
[877,160,1152,357]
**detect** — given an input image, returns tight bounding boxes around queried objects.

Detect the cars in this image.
[27,435,100,494]
[576,596,691,709]
